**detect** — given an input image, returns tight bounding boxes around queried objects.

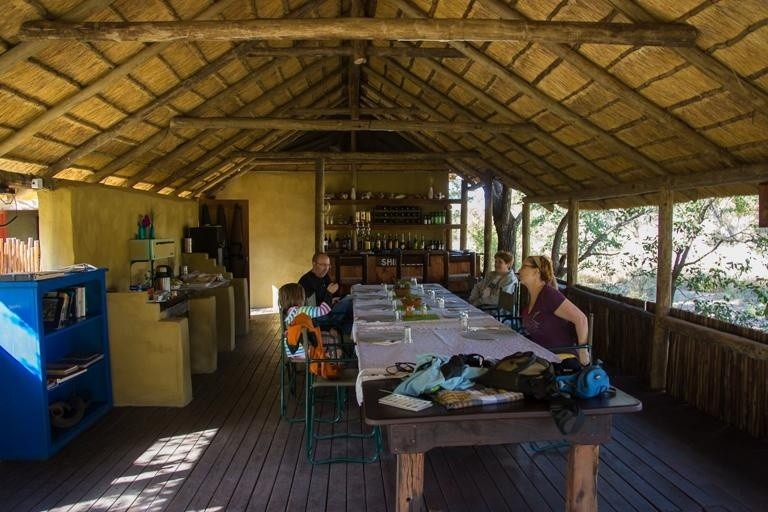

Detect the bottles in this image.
[137,223,155,240]
[155,264,174,293]
[427,184,434,199]
[323,206,448,252]
[350,185,357,200]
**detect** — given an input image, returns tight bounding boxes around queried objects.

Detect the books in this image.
[377,393,433,413]
[42,283,106,391]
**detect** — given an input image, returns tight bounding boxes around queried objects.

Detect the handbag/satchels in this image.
[555,364,610,399]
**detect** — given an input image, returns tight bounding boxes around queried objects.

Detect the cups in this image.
[184,238,192,254]
[181,266,189,276]
[380,277,470,345]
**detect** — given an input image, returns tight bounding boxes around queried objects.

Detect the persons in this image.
[467,249,520,320]
[296,251,353,338]
[276,281,355,369]
[515,254,592,375]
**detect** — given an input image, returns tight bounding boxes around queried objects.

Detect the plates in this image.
[441,310,490,320]
[351,283,405,346]
[463,328,517,341]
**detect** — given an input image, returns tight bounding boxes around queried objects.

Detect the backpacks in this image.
[470,350,559,396]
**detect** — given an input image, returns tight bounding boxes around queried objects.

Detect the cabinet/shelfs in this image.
[321,199,450,275]
[107,277,248,410]
[0,267,117,461]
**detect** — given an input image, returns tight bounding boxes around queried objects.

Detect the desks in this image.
[360,375,644,512]
